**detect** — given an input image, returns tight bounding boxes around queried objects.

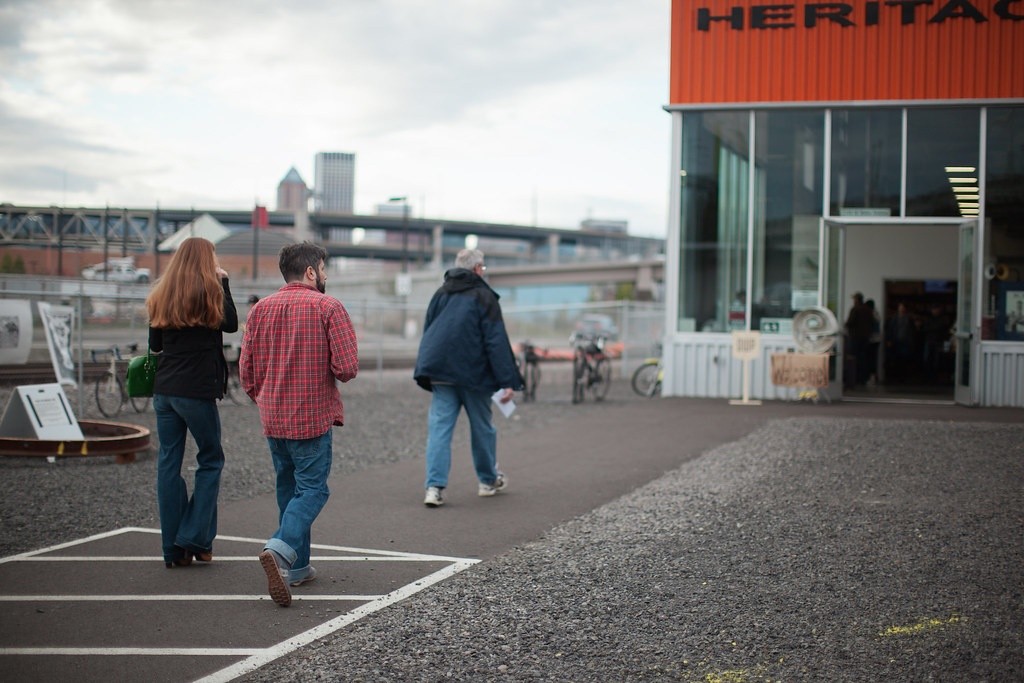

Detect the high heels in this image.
[183,548,213,561]
[166,550,193,567]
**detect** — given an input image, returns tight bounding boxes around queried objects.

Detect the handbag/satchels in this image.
[124,337,161,398]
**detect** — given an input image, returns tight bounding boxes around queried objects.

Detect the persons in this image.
[843,291,917,398]
[148,238,238,567]
[413,248,529,508]
[238,242,358,607]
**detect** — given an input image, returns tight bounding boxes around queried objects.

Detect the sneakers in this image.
[289,564,316,585]
[424,486,448,504]
[258,548,292,607]
[478,470,507,496]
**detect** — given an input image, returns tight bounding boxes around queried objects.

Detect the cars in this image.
[81,261,151,284]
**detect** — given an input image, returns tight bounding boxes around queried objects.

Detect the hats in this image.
[247,295,259,303]
[853,292,863,299]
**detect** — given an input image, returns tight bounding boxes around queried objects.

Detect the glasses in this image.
[476,262,487,271]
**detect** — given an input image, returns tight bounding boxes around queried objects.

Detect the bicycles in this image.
[631,337,665,400]
[519,340,547,404]
[90,342,149,419]
[572,339,613,404]
[223,342,254,406]
